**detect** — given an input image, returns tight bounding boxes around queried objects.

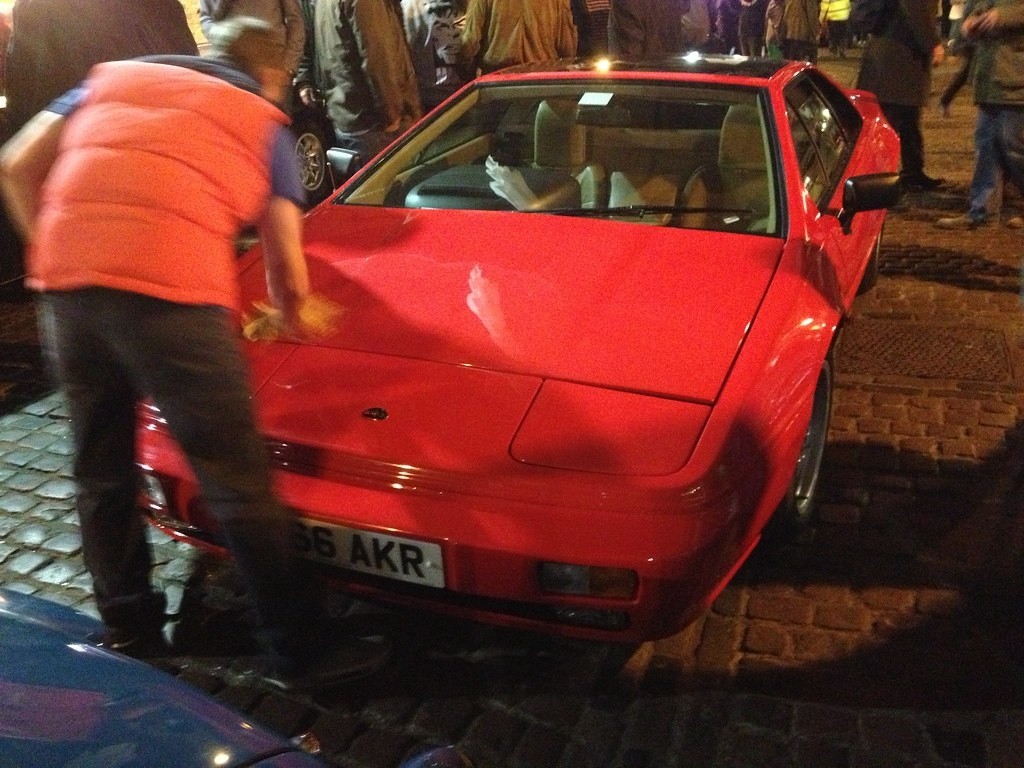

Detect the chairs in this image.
[380,97,837,239]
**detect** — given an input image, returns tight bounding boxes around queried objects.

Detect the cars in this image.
[0,80,331,355]
[0,589,477,768]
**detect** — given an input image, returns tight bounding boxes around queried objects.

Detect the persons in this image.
[0,0,1024,230]
[0,16,396,686]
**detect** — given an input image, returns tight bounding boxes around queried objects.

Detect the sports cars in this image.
[138,54,901,642]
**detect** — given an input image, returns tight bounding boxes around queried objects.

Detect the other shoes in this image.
[937,213,1001,232]
[109,584,168,650]
[1007,214,1024,229]
[268,582,328,655]
[906,175,947,191]
